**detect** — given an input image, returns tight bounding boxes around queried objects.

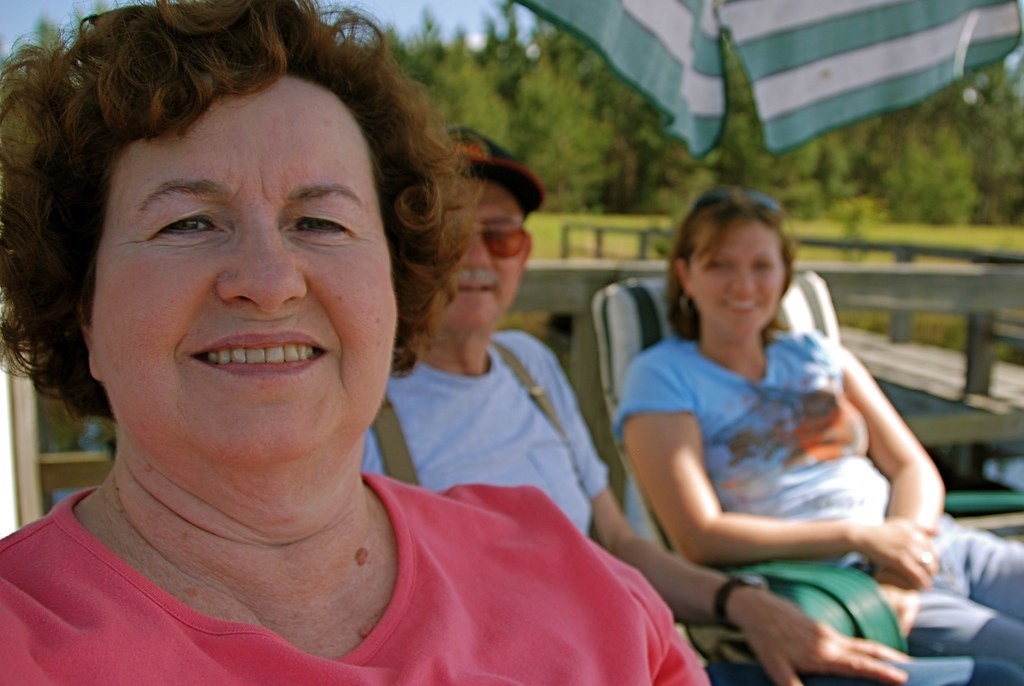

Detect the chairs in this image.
[593,266,1024,686]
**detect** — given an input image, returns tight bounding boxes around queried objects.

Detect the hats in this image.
[444,125,545,214]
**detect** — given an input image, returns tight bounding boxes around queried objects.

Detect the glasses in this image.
[474,221,530,258]
[681,185,782,232]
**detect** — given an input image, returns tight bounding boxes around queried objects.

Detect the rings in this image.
[922,552,935,566]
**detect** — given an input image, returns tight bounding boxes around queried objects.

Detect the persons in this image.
[0,0,716,686]
[358,126,1024,686]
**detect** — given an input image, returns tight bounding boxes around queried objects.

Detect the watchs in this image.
[715,569,767,631]
